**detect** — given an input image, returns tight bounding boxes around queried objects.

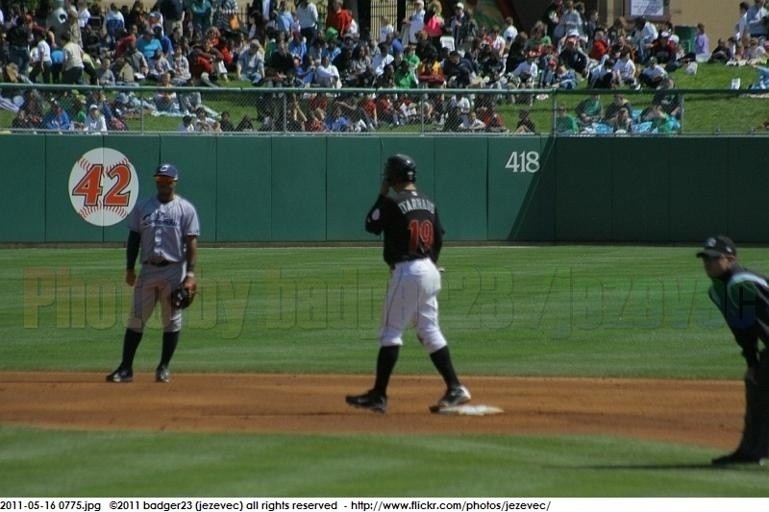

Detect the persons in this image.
[1,1,769,134]
[695,235,769,463]
[346,154,471,413]
[106,165,201,384]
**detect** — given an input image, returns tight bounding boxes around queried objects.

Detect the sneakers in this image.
[713,450,768,466]
[156,366,170,383]
[106,366,132,383]
[430,385,472,415]
[344,389,388,414]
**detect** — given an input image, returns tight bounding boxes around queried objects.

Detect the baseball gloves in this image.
[171,286,196,311]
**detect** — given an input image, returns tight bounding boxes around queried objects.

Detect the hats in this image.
[154,164,177,178]
[89,103,97,111]
[696,235,737,257]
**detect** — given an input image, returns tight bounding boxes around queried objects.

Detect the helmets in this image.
[383,154,416,183]
